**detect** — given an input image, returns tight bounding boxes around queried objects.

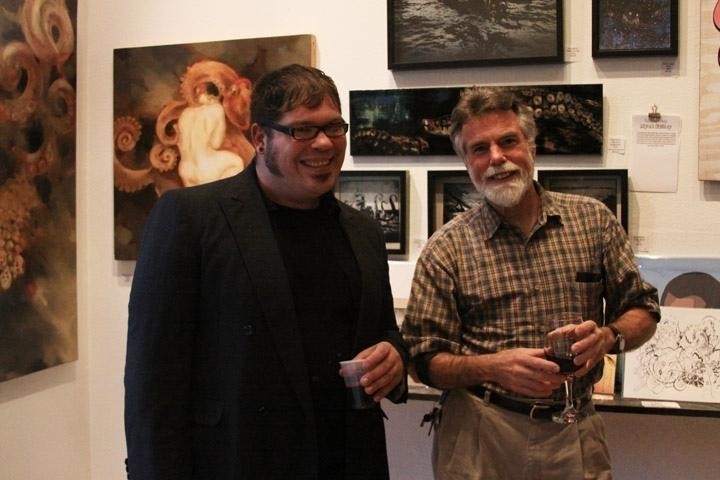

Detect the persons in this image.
[126,65,411,479]
[404,84,662,480]
[170,82,243,187]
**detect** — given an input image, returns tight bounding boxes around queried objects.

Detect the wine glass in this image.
[543,334,588,424]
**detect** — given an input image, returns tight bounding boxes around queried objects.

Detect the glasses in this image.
[266,119,348,141]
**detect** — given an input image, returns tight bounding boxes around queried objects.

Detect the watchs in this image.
[610,325,624,355]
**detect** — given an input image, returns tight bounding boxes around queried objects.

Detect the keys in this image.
[420,412,428,428]
[428,417,433,440]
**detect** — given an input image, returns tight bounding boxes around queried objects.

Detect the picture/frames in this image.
[536,168,628,238]
[385,0,679,72]
[332,167,488,263]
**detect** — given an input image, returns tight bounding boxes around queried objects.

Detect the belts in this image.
[466,383,594,423]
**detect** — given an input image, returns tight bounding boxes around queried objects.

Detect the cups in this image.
[340,359,380,414]
